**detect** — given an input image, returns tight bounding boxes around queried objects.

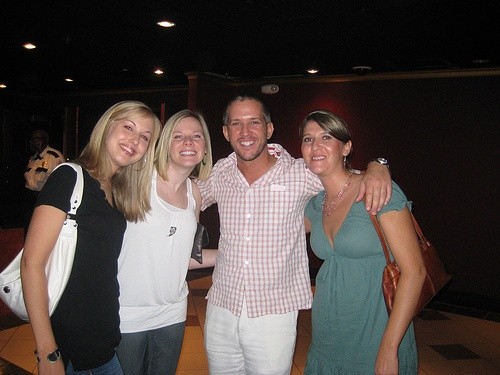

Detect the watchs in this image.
[367,157,391,172]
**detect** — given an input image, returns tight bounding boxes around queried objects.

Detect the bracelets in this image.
[34,346,61,367]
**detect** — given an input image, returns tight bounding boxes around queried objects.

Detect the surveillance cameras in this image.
[261,84,279,94]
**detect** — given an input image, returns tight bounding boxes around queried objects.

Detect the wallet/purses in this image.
[191,221,210,264]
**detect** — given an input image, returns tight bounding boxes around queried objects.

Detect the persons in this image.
[299,110,428,375]
[115,110,219,375]
[23,130,66,239]
[20,100,162,375]
[191,93,392,375]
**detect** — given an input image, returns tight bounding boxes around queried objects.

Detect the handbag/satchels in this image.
[382,237,447,319]
[0,218,79,322]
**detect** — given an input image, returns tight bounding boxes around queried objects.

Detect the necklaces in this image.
[322,172,352,216]
